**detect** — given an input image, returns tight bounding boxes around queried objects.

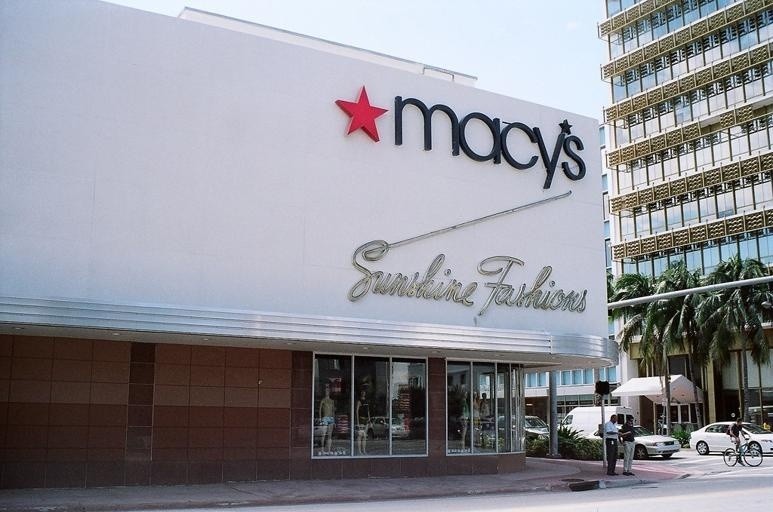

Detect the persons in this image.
[469,391,491,446]
[358,370,385,414]
[317,388,336,453]
[458,389,471,451]
[727,418,747,466]
[617,414,635,475]
[762,418,770,431]
[604,414,620,475]
[355,391,371,456]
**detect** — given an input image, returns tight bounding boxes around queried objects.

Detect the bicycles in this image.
[720,436,762,467]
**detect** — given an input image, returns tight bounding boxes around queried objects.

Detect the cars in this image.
[313,414,408,446]
[688,421,773,457]
[614,425,681,461]
[452,417,503,450]
[497,415,550,456]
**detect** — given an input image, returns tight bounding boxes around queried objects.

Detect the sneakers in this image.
[738,460,745,466]
[734,450,740,456]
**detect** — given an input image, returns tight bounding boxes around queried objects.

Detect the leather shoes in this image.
[606,472,619,476]
[623,472,635,477]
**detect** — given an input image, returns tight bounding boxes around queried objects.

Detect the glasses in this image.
[626,418,634,423]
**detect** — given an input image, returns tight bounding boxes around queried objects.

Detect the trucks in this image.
[557,405,634,457]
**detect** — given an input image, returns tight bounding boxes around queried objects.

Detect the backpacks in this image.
[727,427,732,435]
